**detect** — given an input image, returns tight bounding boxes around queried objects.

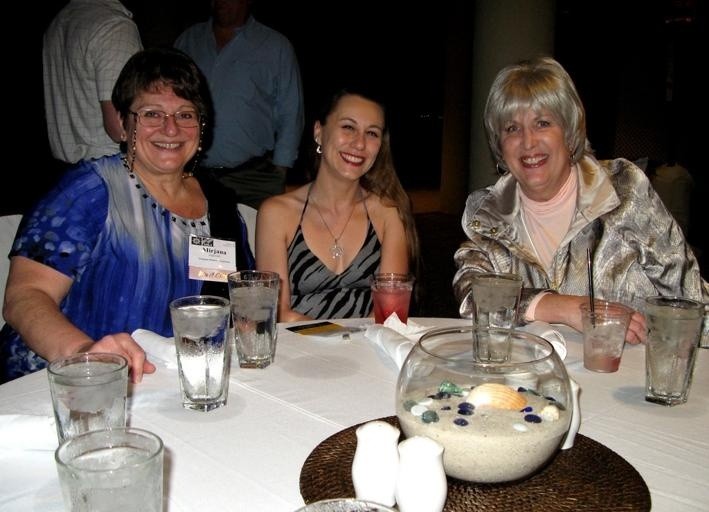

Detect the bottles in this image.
[352,420,447,512]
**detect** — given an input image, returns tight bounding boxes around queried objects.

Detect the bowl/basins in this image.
[469,269,705,409]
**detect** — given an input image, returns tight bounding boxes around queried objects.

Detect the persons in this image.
[453,56,708,346]
[0,45,258,383]
[255,89,426,328]
[169,2,304,211]
[43,2,151,186]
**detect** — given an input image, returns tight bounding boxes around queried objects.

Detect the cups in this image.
[228,272,281,369]
[45,352,128,447]
[169,294,230,412]
[52,427,165,512]
[366,271,417,329]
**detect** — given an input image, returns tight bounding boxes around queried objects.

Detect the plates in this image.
[286,497,399,510]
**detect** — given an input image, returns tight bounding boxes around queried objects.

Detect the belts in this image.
[211,165,244,180]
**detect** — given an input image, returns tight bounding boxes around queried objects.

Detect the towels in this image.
[129,327,183,370]
[364,311,436,378]
[518,317,569,364]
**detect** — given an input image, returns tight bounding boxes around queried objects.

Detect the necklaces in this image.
[307,180,360,260]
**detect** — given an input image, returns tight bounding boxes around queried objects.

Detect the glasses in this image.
[129,108,206,128]
